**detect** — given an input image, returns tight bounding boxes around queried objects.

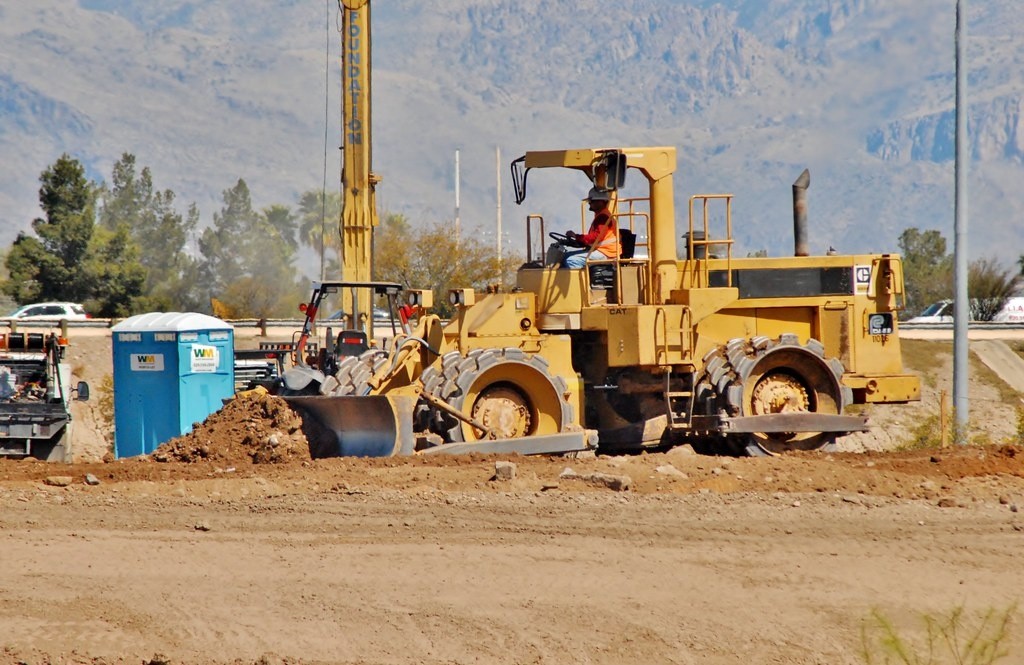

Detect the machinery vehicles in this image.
[249,145,924,461]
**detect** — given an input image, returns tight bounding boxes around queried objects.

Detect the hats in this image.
[583,187,614,201]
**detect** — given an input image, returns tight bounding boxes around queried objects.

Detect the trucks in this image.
[0,333,90,462]
[908,297,1024,324]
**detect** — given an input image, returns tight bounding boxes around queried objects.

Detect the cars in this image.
[330,307,390,318]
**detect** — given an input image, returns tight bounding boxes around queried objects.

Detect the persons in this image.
[563,187,622,268]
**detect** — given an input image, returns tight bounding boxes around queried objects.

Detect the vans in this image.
[0,302,91,327]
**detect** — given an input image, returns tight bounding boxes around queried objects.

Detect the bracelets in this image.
[575,234,578,238]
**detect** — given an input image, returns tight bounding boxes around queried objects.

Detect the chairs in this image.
[333,331,370,372]
[589,229,636,289]
